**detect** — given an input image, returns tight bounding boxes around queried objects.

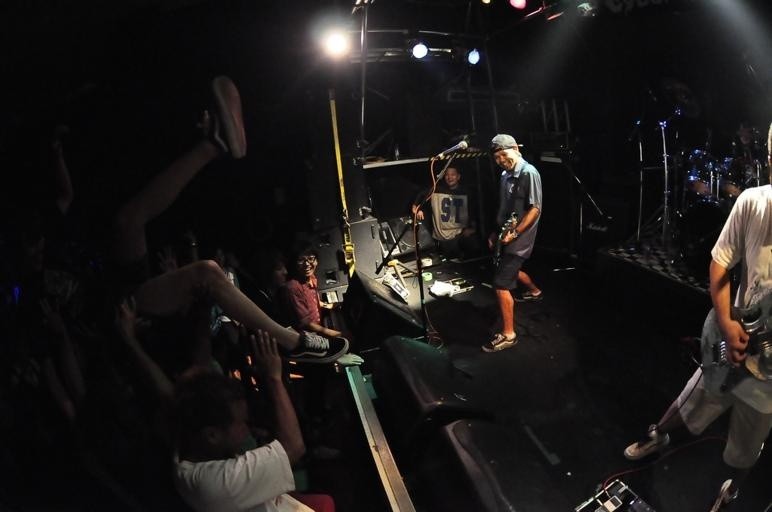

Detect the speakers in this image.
[306,213,576,512]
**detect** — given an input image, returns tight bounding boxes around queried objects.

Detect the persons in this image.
[478,133,543,353]
[2,68,365,511]
[669,121,766,289]
[621,120,771,512]
[412,164,475,266]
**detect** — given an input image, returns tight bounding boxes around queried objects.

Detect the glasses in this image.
[294,256,317,265]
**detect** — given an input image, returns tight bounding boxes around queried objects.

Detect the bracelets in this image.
[512,229,519,239]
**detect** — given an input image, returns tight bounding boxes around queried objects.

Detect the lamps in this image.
[404,37,428,58]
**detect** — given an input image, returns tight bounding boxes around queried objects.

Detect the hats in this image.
[490,133,524,154]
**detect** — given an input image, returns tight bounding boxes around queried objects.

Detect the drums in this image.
[684,150,718,197]
[679,200,733,285]
[719,157,762,198]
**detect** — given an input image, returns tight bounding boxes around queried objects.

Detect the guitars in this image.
[701,290,772,396]
[492,212,518,266]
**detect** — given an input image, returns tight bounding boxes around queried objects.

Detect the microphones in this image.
[436,140,468,160]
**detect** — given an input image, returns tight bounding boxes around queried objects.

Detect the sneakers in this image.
[623,424,670,461]
[481,328,521,353]
[514,289,545,304]
[201,74,248,159]
[710,479,740,511]
[280,328,350,365]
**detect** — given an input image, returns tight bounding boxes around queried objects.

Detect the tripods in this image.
[376,155,472,340]
[351,153,419,289]
